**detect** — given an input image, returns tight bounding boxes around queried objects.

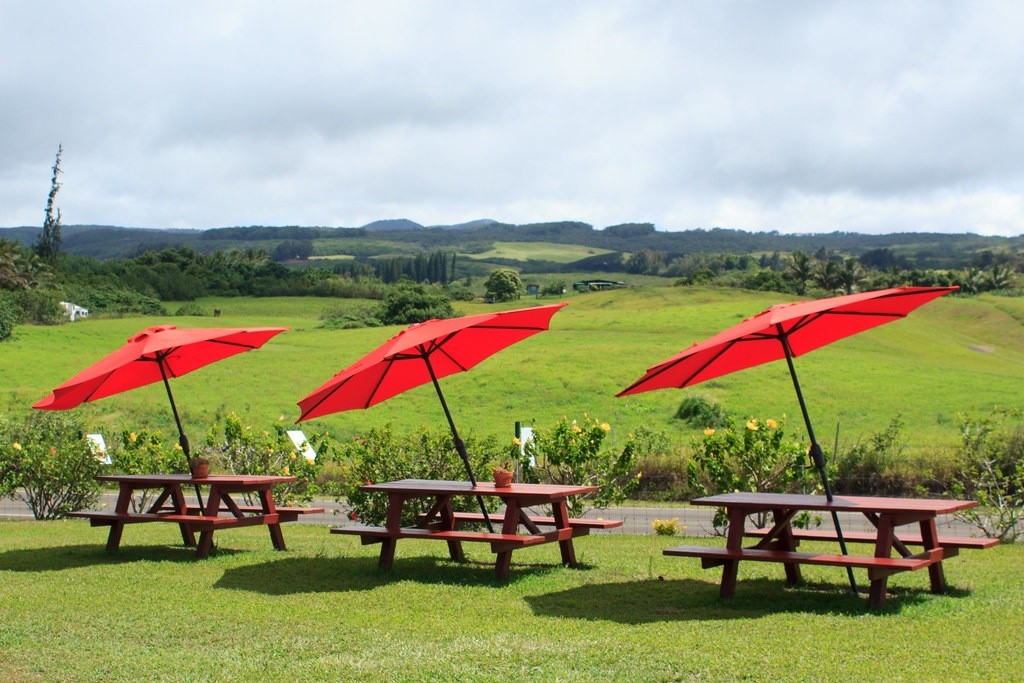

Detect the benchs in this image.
[663,528,999,611]
[328,511,623,580]
[67,504,324,557]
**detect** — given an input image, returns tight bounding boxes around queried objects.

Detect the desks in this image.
[692,492,978,612]
[98,474,297,558]
[360,478,598,581]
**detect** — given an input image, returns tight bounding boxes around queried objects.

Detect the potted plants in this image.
[494,461,514,488]
[191,453,211,479]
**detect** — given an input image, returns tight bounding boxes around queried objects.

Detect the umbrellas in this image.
[293,301,570,553]
[615,286,962,599]
[32,324,292,518]
